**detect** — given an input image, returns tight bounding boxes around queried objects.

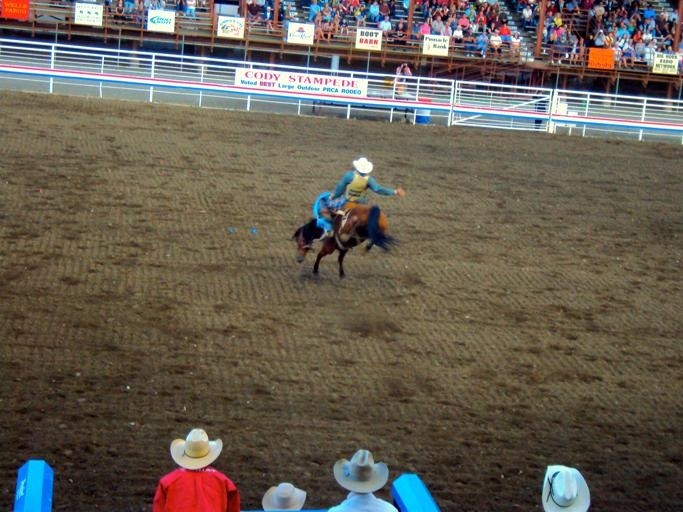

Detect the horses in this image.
[291,205,400,282]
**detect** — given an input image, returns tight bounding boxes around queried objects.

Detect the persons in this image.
[151,429,242,512]
[530,91,548,130]
[50,0,683,80]
[325,448,402,512]
[393,61,413,97]
[314,157,406,241]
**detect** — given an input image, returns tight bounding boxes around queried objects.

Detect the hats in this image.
[169,428,223,471]
[262,483,306,511]
[353,158,373,174]
[542,465,590,511]
[334,450,389,493]
